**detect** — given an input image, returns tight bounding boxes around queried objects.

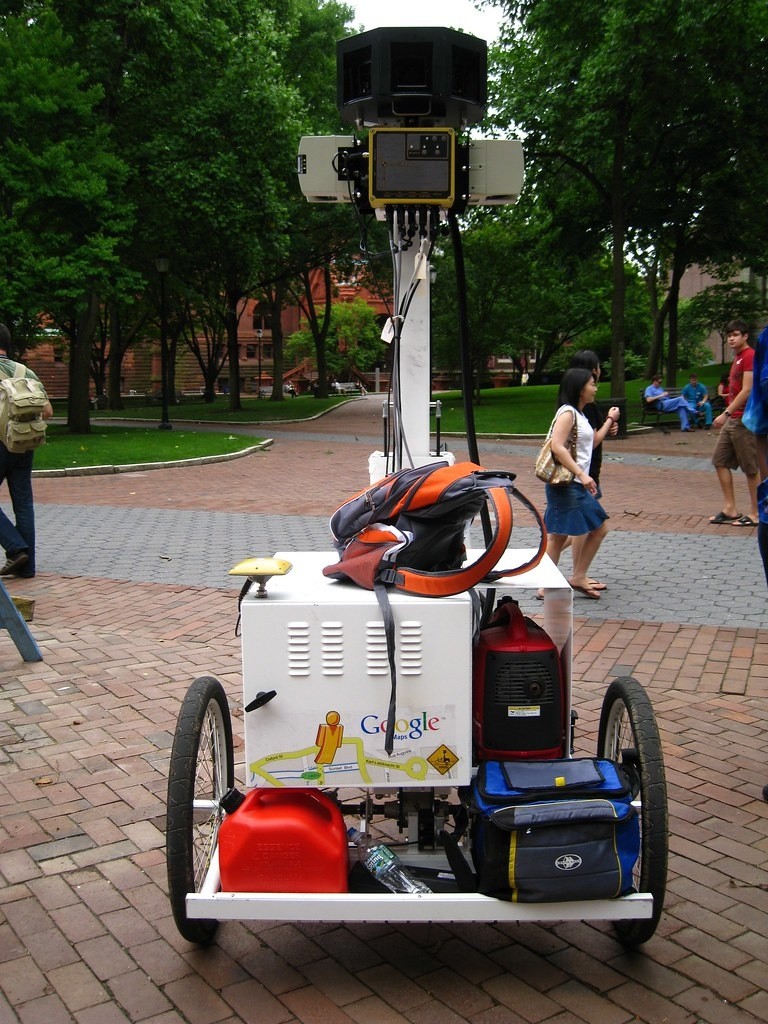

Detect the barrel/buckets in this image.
[218,787,349,893]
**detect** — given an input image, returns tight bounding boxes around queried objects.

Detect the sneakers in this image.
[1,553,37,578]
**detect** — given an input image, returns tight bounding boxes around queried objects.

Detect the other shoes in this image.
[698,423,704,429]
[705,425,710,430]
[685,428,695,432]
[696,412,705,418]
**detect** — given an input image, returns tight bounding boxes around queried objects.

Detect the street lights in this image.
[257,329,263,398]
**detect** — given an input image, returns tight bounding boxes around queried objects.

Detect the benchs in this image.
[181,390,206,400]
[329,382,367,398]
[259,385,294,399]
[639,386,727,436]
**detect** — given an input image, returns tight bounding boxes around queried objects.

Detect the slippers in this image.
[536,595,544,600]
[587,579,607,590]
[709,511,760,526]
[567,581,600,599]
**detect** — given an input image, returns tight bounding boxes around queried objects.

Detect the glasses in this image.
[656,380,662,384]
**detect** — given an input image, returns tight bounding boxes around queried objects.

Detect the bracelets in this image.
[606,414,615,425]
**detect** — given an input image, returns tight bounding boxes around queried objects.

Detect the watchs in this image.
[724,409,732,417]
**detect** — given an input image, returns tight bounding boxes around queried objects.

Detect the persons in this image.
[0,322,54,578]
[520,368,529,386]
[355,378,368,396]
[741,325,768,803]
[709,319,760,527]
[285,380,299,398]
[644,372,730,432]
[331,378,347,397]
[536,348,620,599]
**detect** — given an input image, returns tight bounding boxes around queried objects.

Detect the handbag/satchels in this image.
[446,757,641,903]
[535,405,579,485]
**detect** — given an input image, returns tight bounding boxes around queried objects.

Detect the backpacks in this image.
[321,461,548,600]
[0,362,47,454]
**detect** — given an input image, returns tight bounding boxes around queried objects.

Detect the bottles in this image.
[347,826,433,893]
